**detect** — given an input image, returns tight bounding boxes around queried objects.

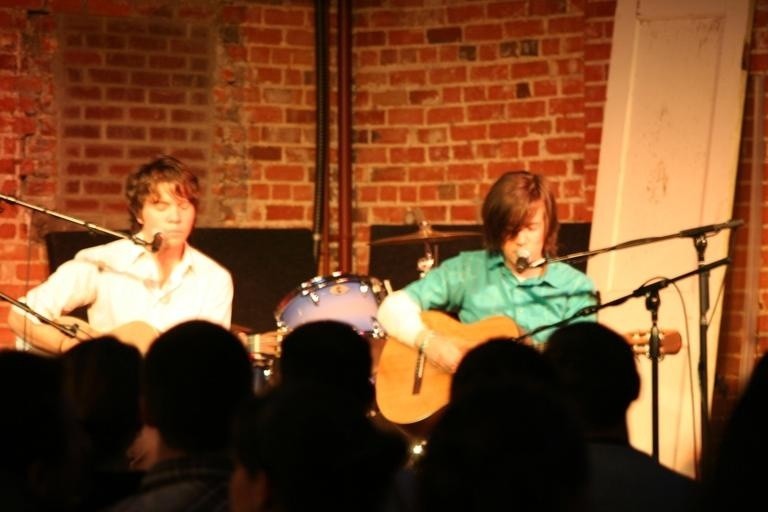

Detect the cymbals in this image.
[366,219,485,246]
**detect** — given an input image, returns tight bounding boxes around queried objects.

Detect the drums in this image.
[272,270,392,374]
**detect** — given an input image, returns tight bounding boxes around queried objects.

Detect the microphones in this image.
[515,249,532,273]
[151,225,165,250]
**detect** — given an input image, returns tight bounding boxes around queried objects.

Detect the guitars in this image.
[28,317,292,367]
[375,308,682,434]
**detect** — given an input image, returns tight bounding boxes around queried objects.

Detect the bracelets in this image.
[416,330,434,356]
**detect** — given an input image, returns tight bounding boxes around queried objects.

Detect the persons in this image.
[1,321,768,512]
[374,169,598,378]
[3,155,234,359]
